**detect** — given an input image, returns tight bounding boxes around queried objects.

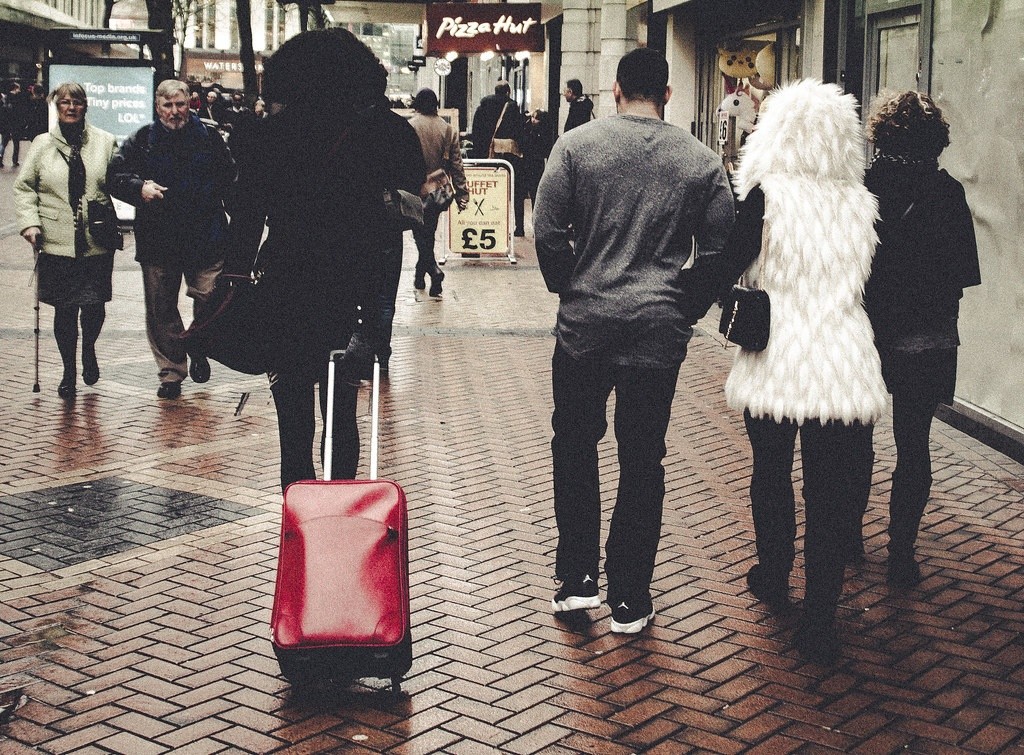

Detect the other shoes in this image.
[13,163,20,167]
[850,531,867,559]
[56,371,79,397]
[81,345,100,385]
[0,155,6,168]
[746,561,791,604]
[514,230,525,236]
[428,264,445,298]
[412,275,425,290]
[884,553,923,588]
[793,622,841,667]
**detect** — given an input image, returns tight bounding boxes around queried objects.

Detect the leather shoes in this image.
[187,348,214,385]
[156,380,183,397]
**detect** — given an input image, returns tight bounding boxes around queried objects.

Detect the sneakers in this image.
[605,593,655,636]
[547,569,603,614]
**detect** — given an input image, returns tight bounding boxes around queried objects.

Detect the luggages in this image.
[270,349,413,694]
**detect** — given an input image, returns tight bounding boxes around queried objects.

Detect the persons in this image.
[473,78,525,238]
[560,79,594,135]
[400,89,469,295]
[848,90,983,595]
[11,81,121,393]
[105,80,237,397]
[178,26,428,496]
[522,110,556,212]
[531,49,745,630]
[0,82,49,168]
[686,74,889,664]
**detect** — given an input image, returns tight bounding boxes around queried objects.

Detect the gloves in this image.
[339,335,377,384]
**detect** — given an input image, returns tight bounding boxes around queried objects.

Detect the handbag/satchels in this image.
[180,273,271,376]
[414,168,461,216]
[382,186,426,233]
[86,197,125,250]
[717,281,772,353]
[485,133,527,170]
